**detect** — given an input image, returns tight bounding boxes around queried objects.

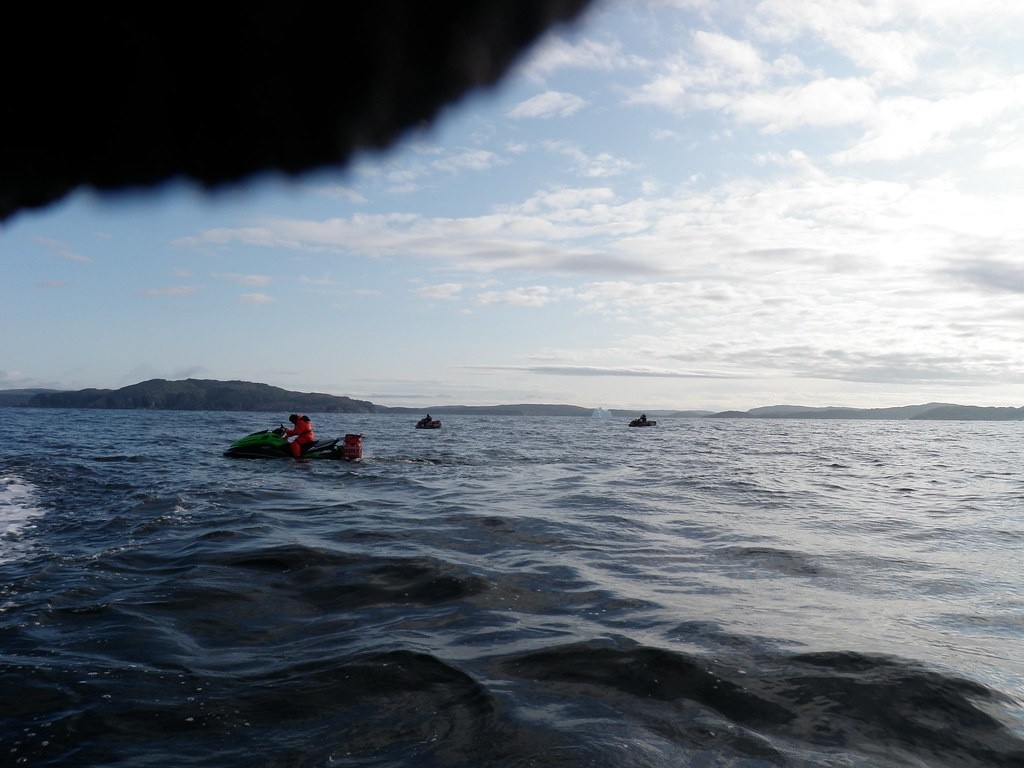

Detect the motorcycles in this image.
[415,419,441,429]
[629,419,656,427]
[227,423,364,462]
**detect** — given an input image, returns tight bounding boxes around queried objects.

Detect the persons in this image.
[284,414,314,459]
[640,414,646,422]
[423,414,432,423]
